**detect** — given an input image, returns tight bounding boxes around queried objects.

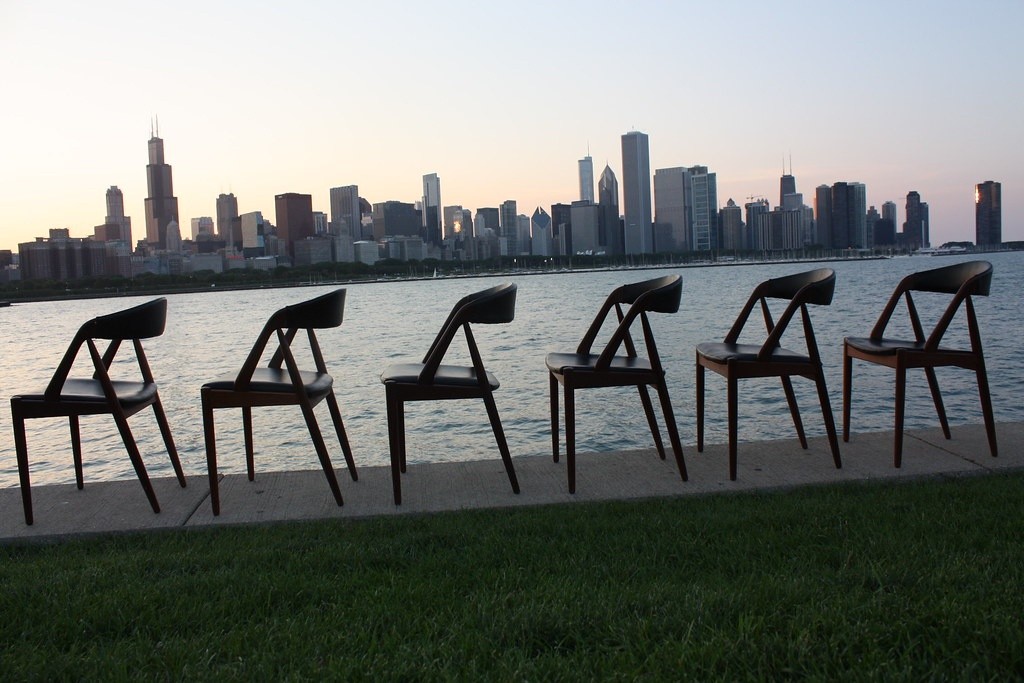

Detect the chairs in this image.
[382,282,520,505]
[695,268,842,481]
[9,297,188,526]
[843,260,998,468]
[200,288,358,516]
[545,274,688,493]
